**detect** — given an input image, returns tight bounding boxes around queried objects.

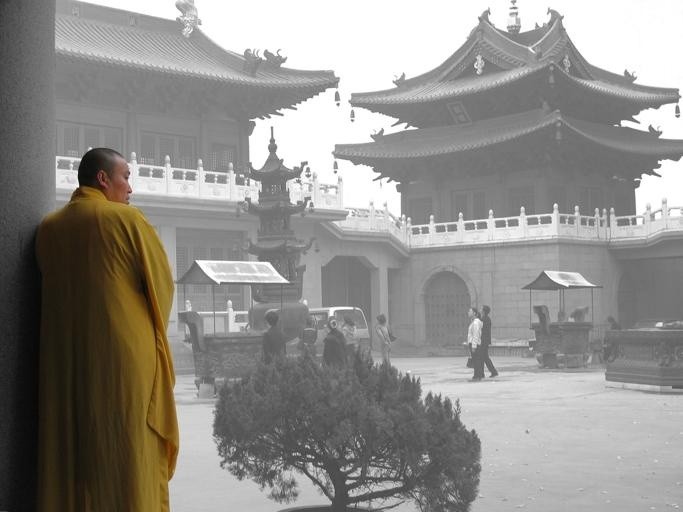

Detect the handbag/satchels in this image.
[466,358,472,369]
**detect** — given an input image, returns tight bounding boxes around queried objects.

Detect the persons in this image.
[375,314,391,364]
[32,148,180,512]
[478,305,499,378]
[602,314,623,363]
[467,307,483,381]
[302,315,359,370]
[260,311,286,365]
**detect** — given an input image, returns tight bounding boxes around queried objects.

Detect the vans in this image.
[301,307,370,362]
